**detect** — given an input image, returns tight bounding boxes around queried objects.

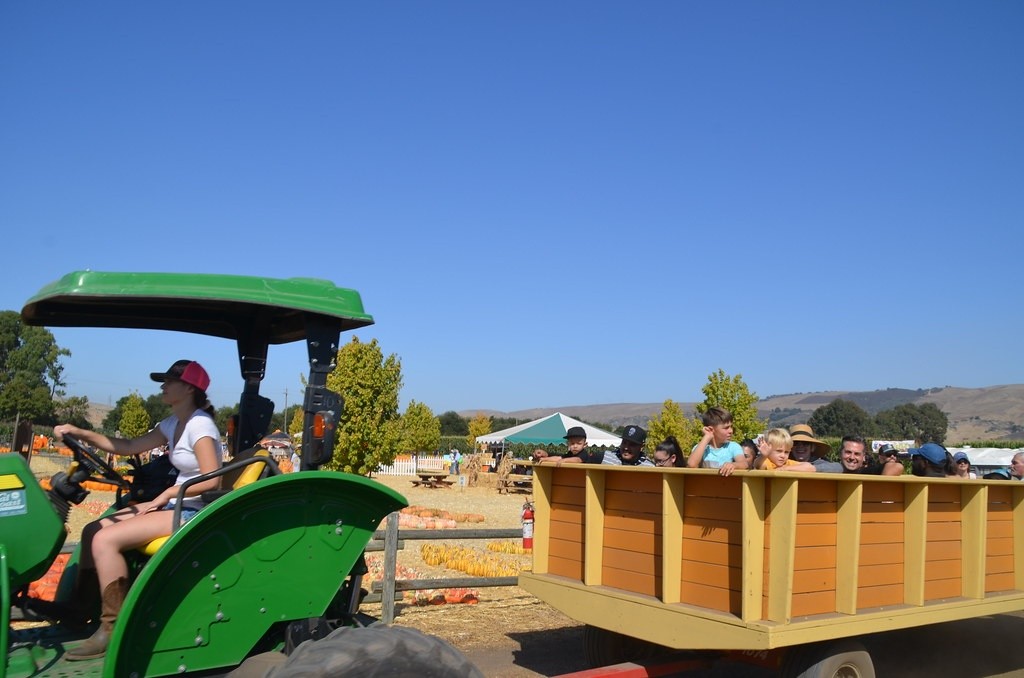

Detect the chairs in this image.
[129,445,271,558]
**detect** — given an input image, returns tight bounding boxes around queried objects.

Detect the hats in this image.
[150,360,210,391]
[907,443,947,466]
[788,424,830,462]
[879,444,899,455]
[954,452,969,462]
[619,425,647,444]
[983,469,1011,480]
[563,427,587,439]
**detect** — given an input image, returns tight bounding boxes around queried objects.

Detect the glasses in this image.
[882,452,898,457]
[911,455,924,461]
[653,453,673,465]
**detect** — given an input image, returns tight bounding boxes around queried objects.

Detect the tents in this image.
[476,411,623,461]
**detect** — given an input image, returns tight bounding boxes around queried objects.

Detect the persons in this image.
[787,424,844,473]
[654,436,685,467]
[907,442,947,477]
[29,360,223,661]
[739,439,758,469]
[983,452,1024,480]
[940,445,977,480]
[538,427,589,465]
[140,442,169,465]
[686,407,749,477]
[839,435,904,476]
[878,444,902,465]
[753,428,817,472]
[433,445,463,476]
[591,424,656,467]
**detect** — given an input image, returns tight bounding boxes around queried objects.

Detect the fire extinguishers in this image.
[522,497,535,549]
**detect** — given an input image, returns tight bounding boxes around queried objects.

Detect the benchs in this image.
[496,484,532,494]
[408,479,456,489]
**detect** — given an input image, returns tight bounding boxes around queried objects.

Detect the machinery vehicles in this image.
[0,269,1024,678]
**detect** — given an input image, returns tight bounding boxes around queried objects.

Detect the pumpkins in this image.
[360,506,534,606]
[39,474,134,493]
[0,446,39,454]
[59,445,74,455]
[17,553,72,601]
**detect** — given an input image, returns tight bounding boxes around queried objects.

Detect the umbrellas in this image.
[266,428,290,449]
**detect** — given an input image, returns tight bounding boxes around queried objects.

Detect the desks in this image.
[416,473,450,486]
[499,478,533,494]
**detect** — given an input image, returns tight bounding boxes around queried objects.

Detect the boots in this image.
[65,576,130,661]
[33,579,98,643]
